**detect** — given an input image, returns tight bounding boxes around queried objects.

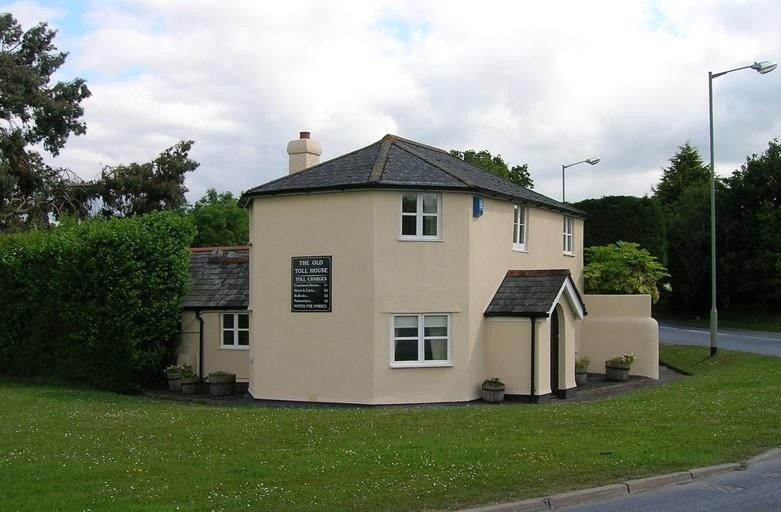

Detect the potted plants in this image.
[482,378,505,402]
[181,371,236,396]
[575,351,590,386]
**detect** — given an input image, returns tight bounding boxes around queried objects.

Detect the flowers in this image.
[163,364,186,373]
[608,351,636,367]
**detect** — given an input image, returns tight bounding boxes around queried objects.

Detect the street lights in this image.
[706,61,776,350]
[561,158,599,204]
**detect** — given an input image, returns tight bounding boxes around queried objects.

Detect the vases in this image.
[605,360,630,381]
[165,372,182,391]
